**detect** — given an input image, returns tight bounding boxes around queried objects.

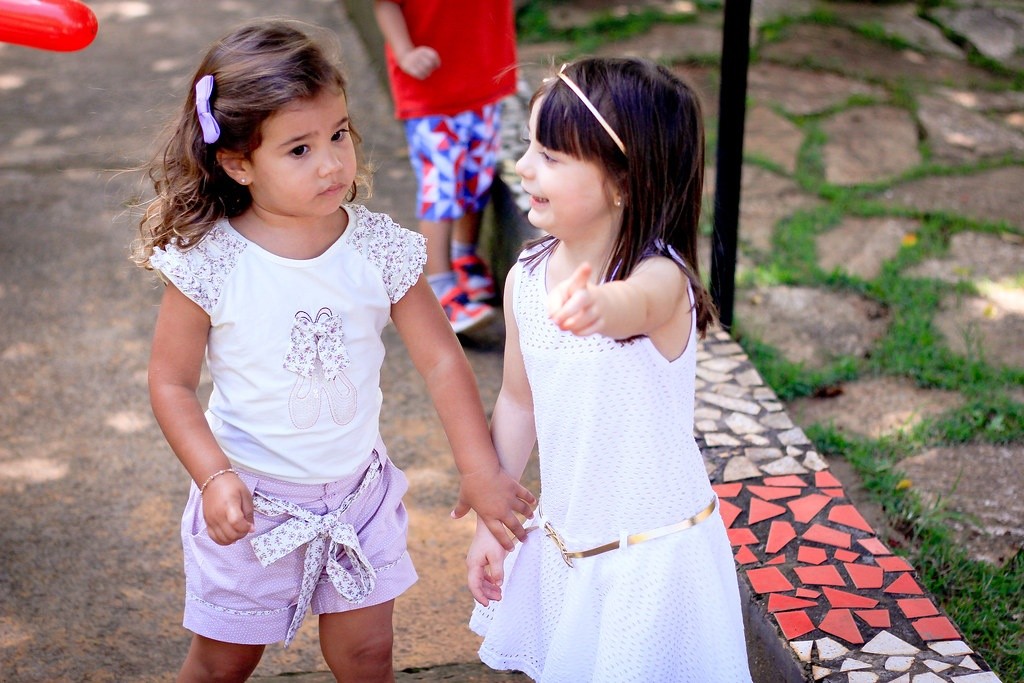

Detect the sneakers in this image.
[451,255,496,301]
[439,288,496,335]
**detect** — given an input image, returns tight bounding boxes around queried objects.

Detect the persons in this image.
[371,0,521,336]
[458,51,756,682]
[135,16,538,683]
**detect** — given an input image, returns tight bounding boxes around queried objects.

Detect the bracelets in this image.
[199,467,238,496]
